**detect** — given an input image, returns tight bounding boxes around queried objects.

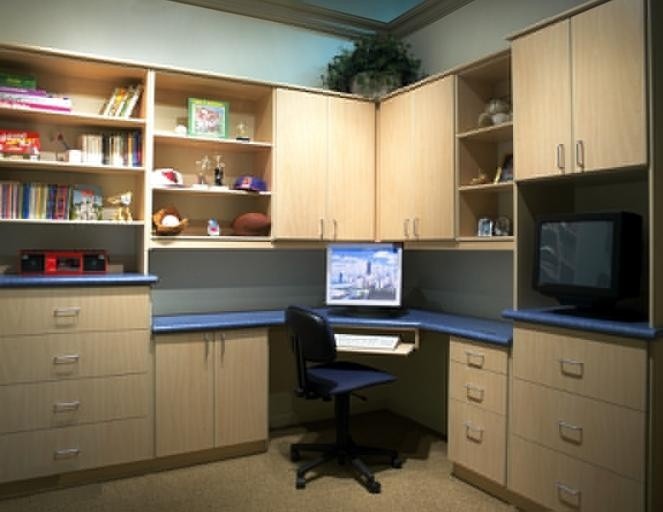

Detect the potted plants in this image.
[318,30,429,98]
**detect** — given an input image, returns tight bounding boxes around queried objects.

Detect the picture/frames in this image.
[185,96,228,141]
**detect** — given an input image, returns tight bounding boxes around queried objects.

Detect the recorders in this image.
[18,249,108,277]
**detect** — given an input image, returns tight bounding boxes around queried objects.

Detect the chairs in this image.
[288,305,407,491]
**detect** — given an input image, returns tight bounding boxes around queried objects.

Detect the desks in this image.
[159,305,515,350]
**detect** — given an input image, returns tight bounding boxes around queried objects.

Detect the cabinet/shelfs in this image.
[156,64,272,251]
[274,83,377,250]
[507,0,663,512]
[153,329,293,477]
[456,50,512,249]
[1,41,154,498]
[377,70,457,250]
[446,335,506,494]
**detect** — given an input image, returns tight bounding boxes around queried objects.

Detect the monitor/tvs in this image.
[531,211,644,319]
[323,241,406,309]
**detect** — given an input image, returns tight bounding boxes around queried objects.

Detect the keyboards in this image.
[332,331,401,350]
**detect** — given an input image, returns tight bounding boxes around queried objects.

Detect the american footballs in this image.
[232,212,272,237]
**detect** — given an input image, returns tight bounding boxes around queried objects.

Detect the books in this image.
[0,178,102,222]
[56,130,142,166]
[98,81,143,117]
[1,127,40,160]
[1,71,73,114]
